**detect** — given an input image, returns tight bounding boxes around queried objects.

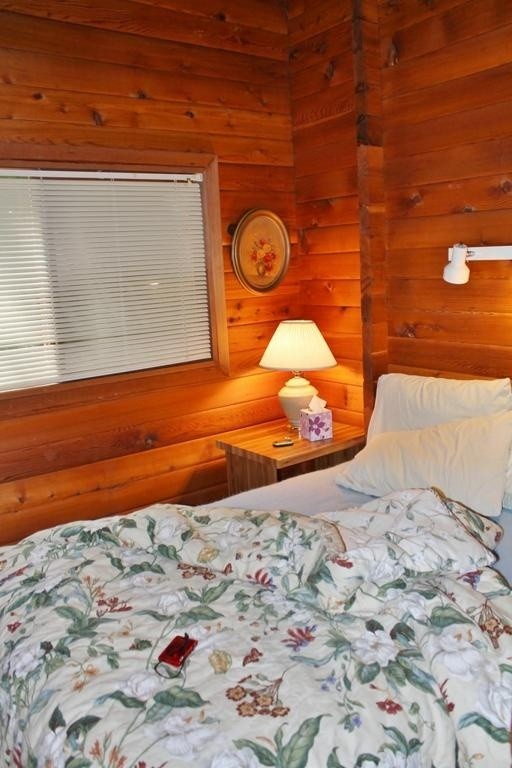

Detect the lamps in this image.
[443,242,512,286]
[257,320,339,431]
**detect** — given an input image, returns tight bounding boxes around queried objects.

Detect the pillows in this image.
[336,412,512,516]
[366,372,512,445]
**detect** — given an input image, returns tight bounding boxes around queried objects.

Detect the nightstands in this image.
[213,419,366,497]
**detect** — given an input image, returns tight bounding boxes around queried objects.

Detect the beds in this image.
[1,363,512,767]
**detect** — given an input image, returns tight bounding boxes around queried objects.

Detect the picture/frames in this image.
[230,208,292,296]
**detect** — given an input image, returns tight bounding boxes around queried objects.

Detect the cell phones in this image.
[158,635,198,667]
[273,439,294,446]
